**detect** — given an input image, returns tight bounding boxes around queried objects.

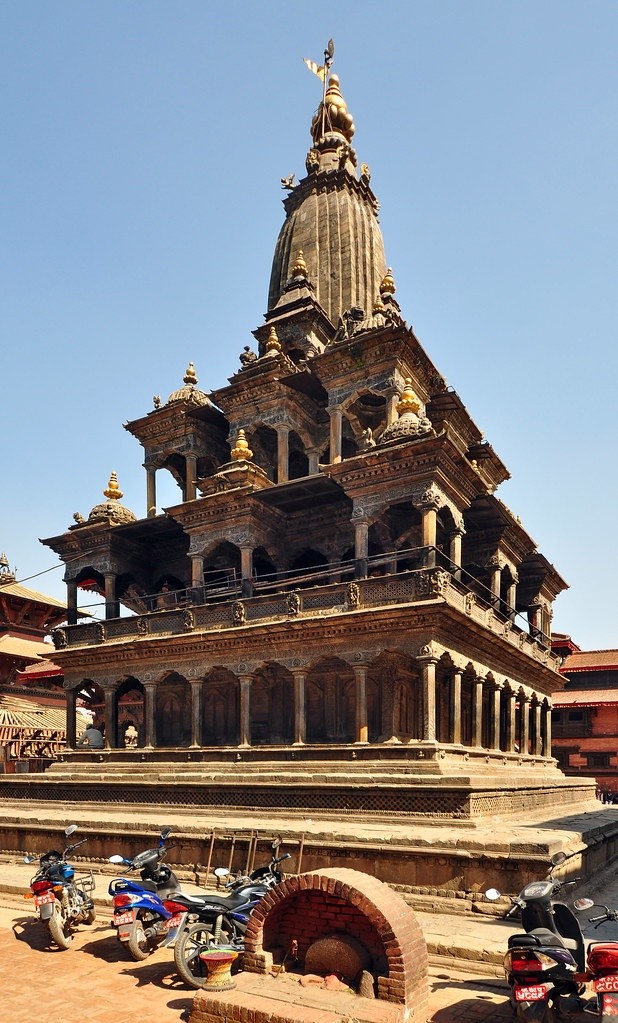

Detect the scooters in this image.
[483,852,588,1023]
[573,897,618,1022]
[109,828,183,961]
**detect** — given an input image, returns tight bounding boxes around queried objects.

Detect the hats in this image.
[86,724,95,730]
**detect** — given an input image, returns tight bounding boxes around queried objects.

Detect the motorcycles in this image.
[170,836,290,988]
[24,824,95,950]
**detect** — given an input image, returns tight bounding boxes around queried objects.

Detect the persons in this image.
[76,724,104,748]
[600,788,618,804]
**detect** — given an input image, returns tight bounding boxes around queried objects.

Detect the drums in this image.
[199,950,238,992]
